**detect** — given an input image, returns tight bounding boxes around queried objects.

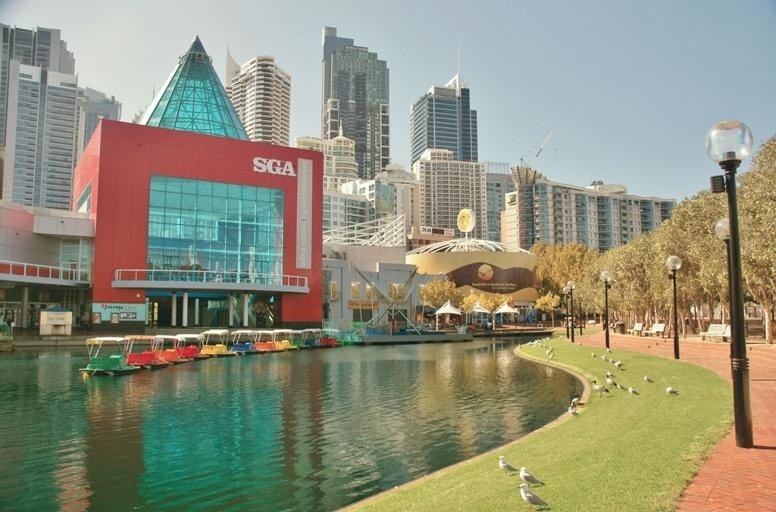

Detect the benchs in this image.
[699,323,728,343]
[625,322,667,338]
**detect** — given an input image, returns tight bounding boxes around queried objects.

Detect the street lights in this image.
[665,254,683,360]
[704,119,755,448]
[562,280,584,343]
[598,270,611,352]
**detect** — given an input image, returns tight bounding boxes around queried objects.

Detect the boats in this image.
[78,326,342,377]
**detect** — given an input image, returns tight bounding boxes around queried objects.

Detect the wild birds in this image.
[497,455,550,511]
[567,393,580,415]
[518,336,556,361]
[665,386,680,397]
[589,347,654,398]
